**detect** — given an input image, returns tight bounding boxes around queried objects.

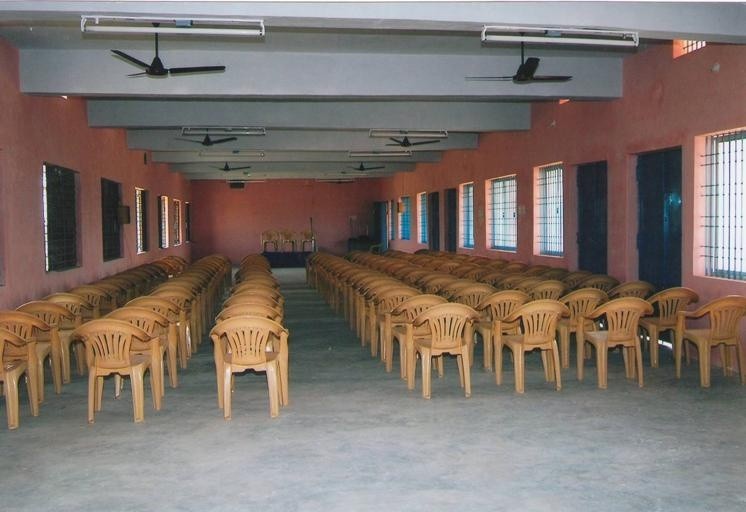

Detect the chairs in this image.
[1,254,289,430]
[257,228,317,253]
[676,293,745,388]
[306,247,699,400]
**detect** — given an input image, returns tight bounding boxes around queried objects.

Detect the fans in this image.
[114,23,223,79]
[464,30,575,87]
[388,131,443,150]
[176,126,239,147]
[211,161,387,187]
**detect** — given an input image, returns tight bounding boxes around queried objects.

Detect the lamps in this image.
[347,147,414,159]
[197,150,266,160]
[183,122,264,137]
[369,130,450,140]
[75,14,269,38]
[483,26,642,53]
